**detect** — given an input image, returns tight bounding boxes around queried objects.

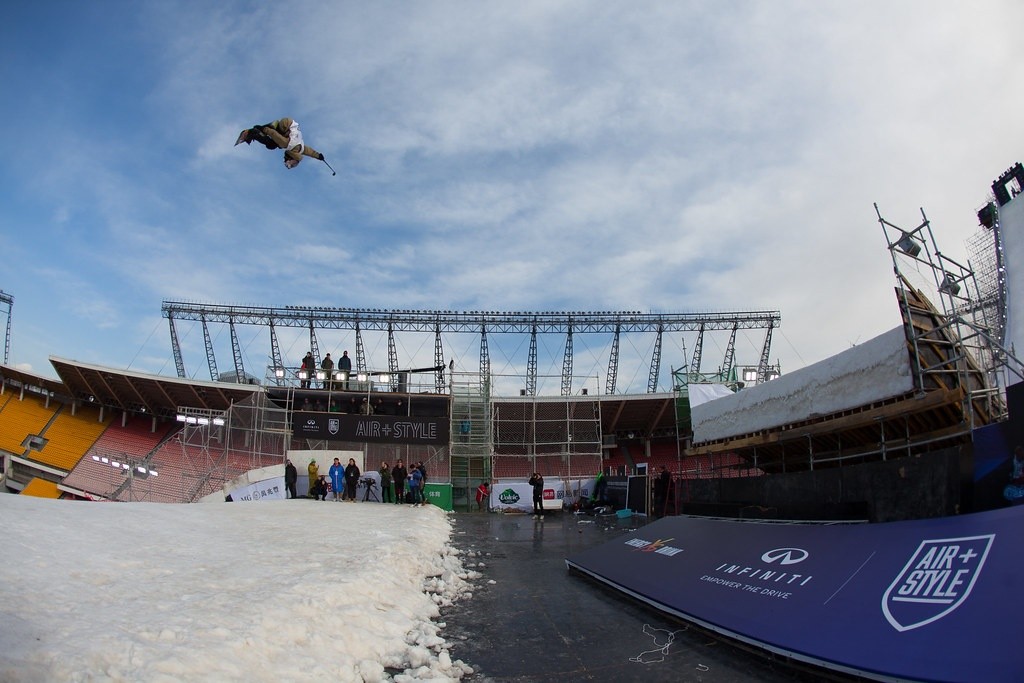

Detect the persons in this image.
[328,457,344,501]
[337,351,351,389]
[406,463,421,506]
[379,461,393,503]
[657,463,672,505]
[313,474,327,501]
[285,458,296,498]
[528,472,544,519]
[416,460,426,505]
[459,414,469,443]
[475,481,490,512]
[300,351,314,387]
[391,458,407,504]
[320,353,334,388]
[308,458,319,497]
[344,457,359,502]
[234,116,323,169]
[299,397,406,415]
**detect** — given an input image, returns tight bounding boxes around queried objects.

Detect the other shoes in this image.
[349,498,357,503]
[532,515,538,520]
[411,503,418,508]
[540,515,544,520]
[334,498,342,502]
[421,501,427,506]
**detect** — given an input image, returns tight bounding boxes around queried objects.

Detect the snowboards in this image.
[234,120,280,147]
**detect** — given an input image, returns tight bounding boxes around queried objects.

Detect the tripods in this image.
[361,486,380,503]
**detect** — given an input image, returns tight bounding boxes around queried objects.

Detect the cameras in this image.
[533,473,538,479]
[360,477,376,486]
[321,477,325,481]
